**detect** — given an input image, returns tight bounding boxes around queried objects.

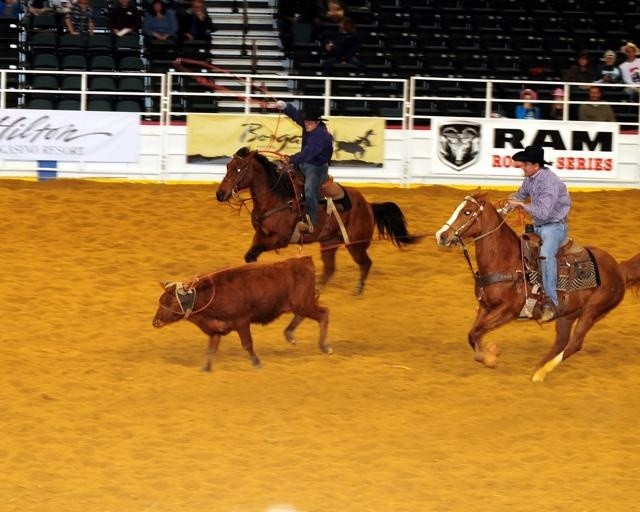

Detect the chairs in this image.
[276,0,640,122]
[0,0,217,111]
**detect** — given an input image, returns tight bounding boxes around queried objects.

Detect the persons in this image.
[286,1,374,77]
[276,101,332,233]
[505,146,571,323]
[513,42,639,120]
[0,0,218,104]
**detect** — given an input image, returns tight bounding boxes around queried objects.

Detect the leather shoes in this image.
[297,220,308,232]
[541,310,554,322]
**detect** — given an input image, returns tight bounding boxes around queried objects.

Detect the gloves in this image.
[275,99,288,110]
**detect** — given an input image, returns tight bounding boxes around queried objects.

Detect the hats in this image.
[512,146,552,165]
[551,88,564,96]
[520,88,537,99]
[297,106,329,121]
[620,41,640,56]
[604,49,616,58]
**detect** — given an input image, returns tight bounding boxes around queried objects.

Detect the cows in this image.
[153,256,333,372]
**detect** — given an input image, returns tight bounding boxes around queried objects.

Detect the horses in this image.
[216,148,434,296]
[436,186,640,382]
